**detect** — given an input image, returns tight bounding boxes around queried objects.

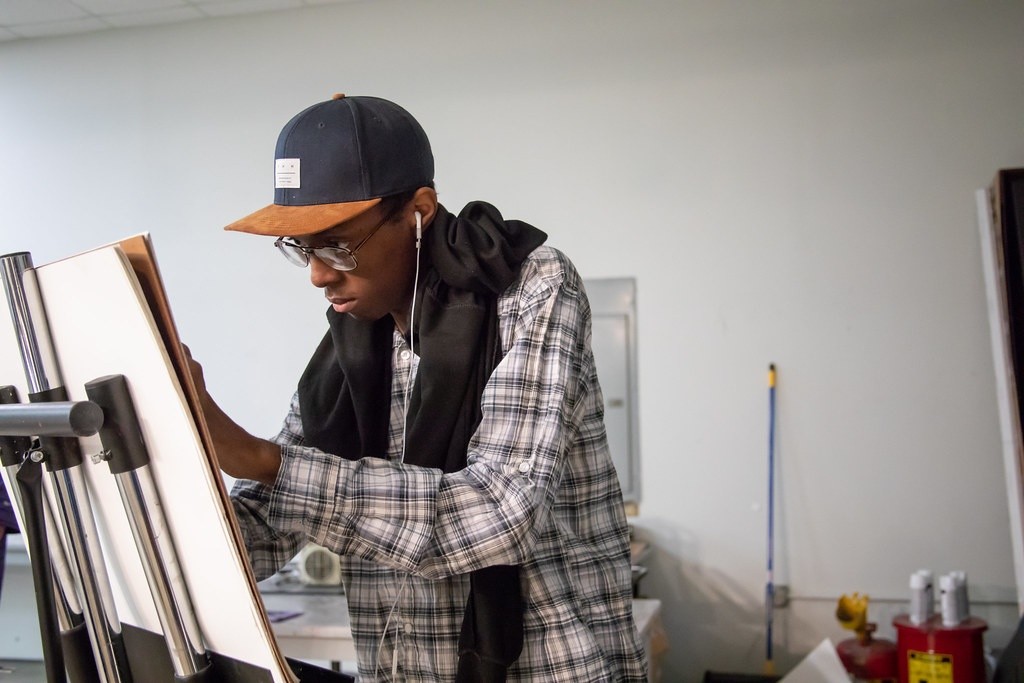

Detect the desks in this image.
[258,593,660,683]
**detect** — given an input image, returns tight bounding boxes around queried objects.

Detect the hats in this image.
[224,94,435,236]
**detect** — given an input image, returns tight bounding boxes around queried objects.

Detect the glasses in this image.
[275,208,395,271]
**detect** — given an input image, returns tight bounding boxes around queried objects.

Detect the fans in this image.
[299,544,340,586]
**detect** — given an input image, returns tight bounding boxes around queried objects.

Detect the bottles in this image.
[910,568,970,627]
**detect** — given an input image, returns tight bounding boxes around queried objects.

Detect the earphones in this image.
[414,211,422,239]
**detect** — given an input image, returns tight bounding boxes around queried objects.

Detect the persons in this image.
[181,95,647,683]
